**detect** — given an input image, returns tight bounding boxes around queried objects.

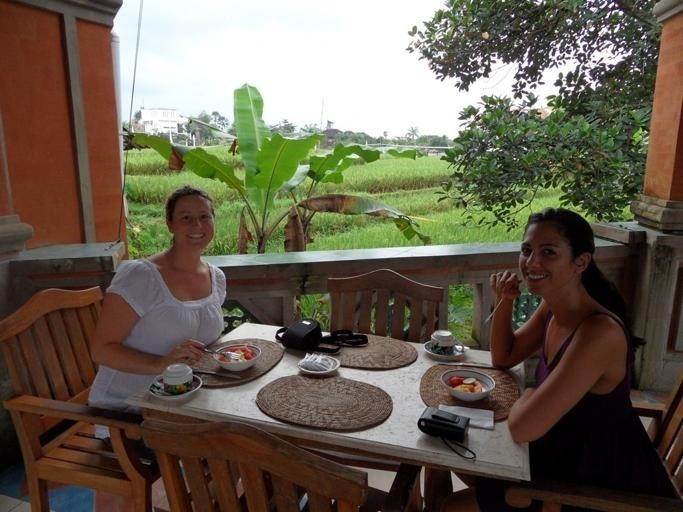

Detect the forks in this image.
[202,348,240,360]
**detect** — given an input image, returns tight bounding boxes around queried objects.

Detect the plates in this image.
[298,356,340,375]
[423,340,466,359]
[148,374,202,400]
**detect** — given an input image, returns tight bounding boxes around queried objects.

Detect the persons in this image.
[86,186,226,457]
[446,208,634,508]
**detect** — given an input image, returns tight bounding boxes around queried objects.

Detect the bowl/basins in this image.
[211,344,261,372]
[441,369,495,402]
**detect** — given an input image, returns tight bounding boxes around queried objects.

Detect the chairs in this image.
[325,267,445,343]
[504,369,682,511]
[0,285,181,510]
[137,409,425,511]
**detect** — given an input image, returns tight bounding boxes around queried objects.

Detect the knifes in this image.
[191,369,241,380]
[438,362,494,369]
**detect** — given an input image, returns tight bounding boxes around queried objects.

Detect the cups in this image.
[153,363,193,395]
[430,330,464,355]
